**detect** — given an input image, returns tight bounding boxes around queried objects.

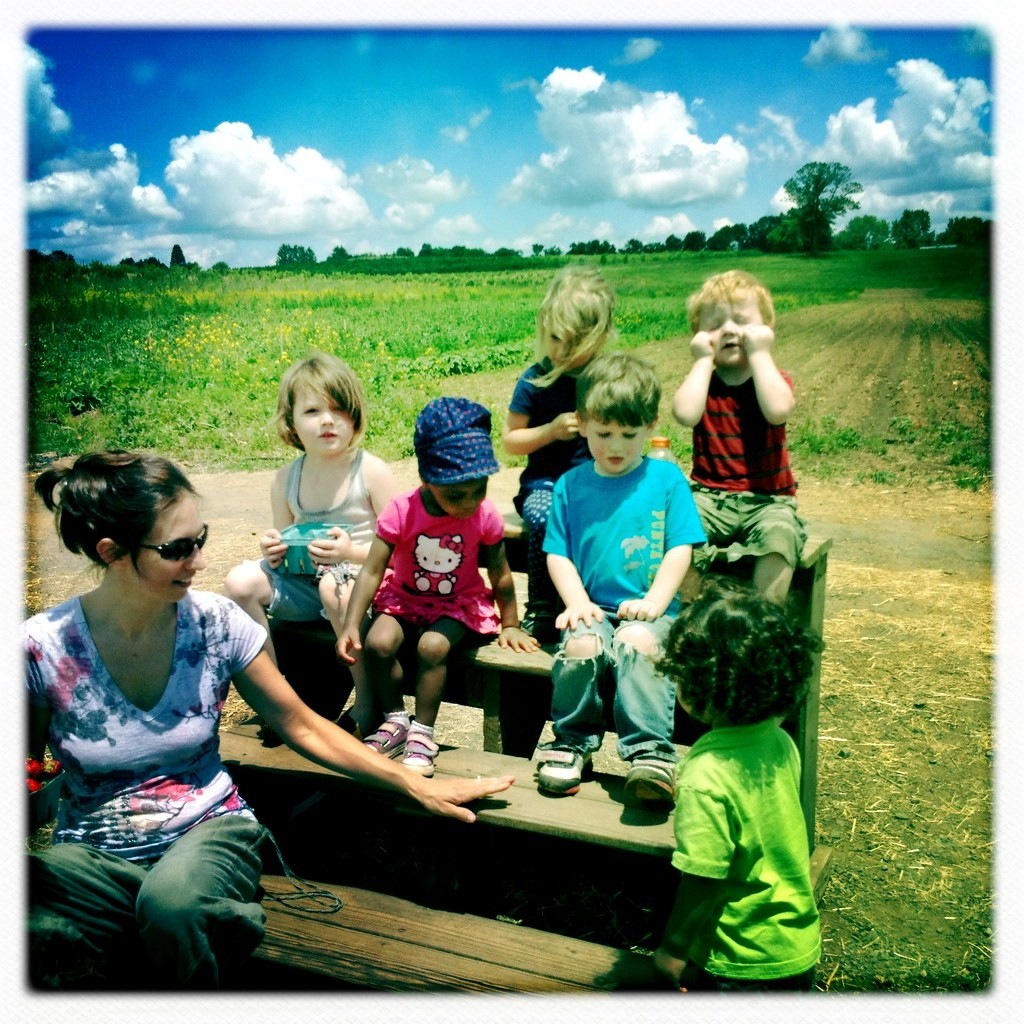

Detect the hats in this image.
[414,397,499,484]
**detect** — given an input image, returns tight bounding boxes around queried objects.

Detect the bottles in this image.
[647,437,676,463]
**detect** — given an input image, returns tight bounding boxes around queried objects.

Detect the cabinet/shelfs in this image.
[216,522,831,994]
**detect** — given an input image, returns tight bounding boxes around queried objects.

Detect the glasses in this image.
[139,524,209,561]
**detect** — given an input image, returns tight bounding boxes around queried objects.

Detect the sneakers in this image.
[400,731,439,776]
[364,721,409,760]
[536,739,592,794]
[624,750,677,813]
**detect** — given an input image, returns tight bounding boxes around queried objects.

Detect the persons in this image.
[219,263,808,812]
[22,445,518,992]
[645,572,827,994]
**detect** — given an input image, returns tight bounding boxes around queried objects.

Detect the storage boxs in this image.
[277,522,353,574]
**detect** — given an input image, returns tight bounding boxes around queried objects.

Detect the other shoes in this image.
[521,601,560,644]
